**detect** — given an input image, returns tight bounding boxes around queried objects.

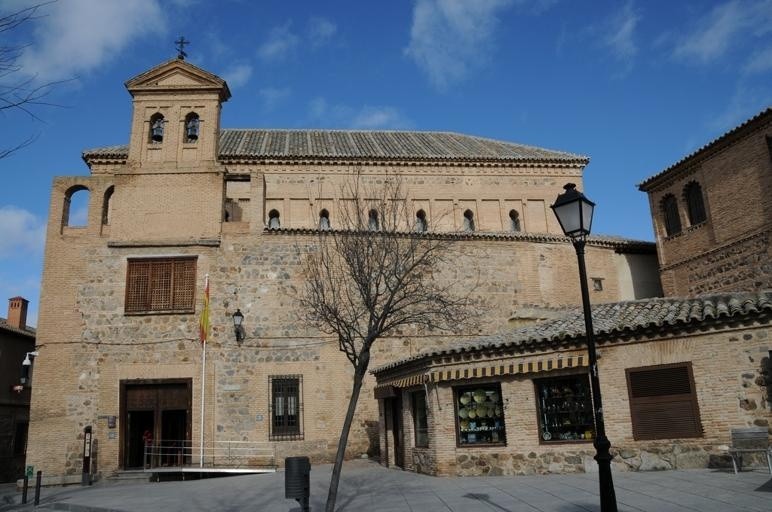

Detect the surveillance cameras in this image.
[21,360,32,368]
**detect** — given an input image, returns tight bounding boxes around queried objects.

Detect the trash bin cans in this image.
[285,456,311,512]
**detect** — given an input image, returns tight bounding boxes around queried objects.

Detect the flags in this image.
[198,278,212,344]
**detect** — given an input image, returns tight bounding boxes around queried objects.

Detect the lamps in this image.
[232,308,245,340]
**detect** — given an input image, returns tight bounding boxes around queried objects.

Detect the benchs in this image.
[728,427,772,475]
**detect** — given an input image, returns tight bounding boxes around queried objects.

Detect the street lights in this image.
[550,183,618,511]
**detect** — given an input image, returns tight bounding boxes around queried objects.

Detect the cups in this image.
[581,434,584,439]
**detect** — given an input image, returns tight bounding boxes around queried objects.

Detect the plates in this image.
[459,389,503,429]
